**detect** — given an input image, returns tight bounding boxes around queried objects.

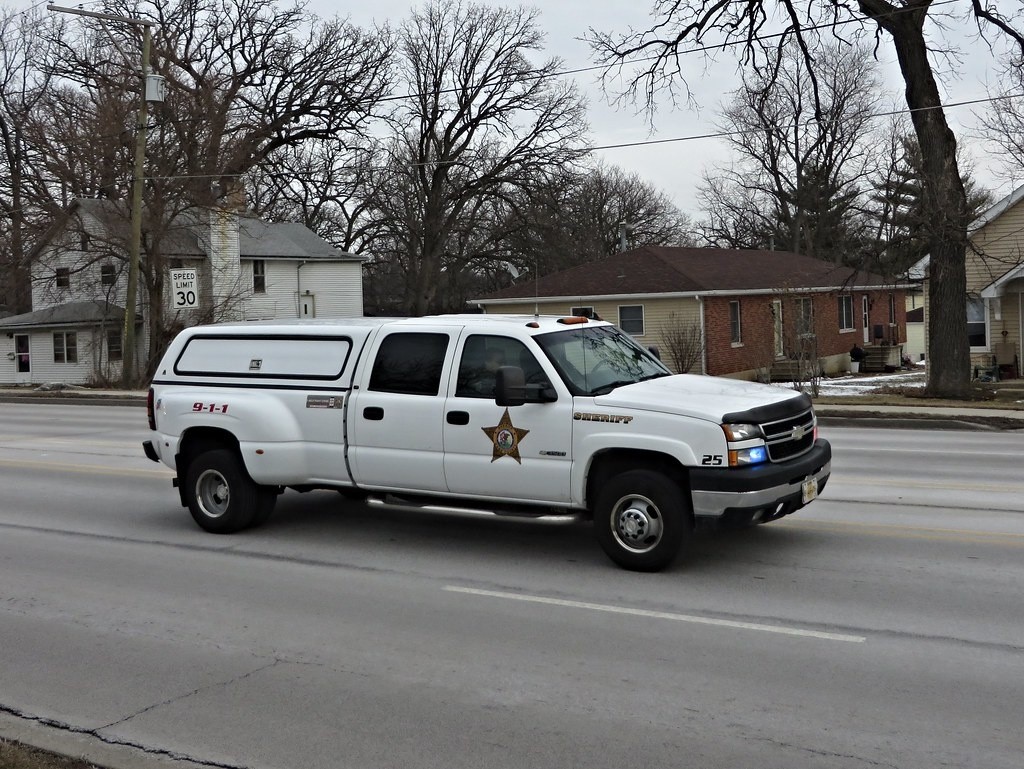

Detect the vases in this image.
[850,362,860,374]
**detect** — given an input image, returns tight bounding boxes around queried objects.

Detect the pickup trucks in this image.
[142,312,833,573]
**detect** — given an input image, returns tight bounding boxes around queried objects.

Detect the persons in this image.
[476,347,506,394]
[525,343,582,385]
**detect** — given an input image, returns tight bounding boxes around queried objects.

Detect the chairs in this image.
[520,349,538,371]
[992,343,1018,380]
